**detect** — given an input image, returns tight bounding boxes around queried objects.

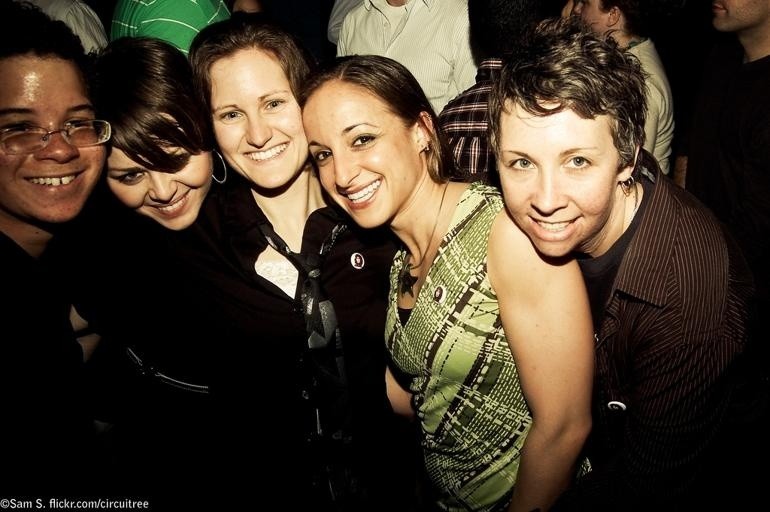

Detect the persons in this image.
[0,0,770,512]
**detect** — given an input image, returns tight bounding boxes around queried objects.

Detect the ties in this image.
[260,218,351,445]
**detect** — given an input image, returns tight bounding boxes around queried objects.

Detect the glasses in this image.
[2,118,111,154]
